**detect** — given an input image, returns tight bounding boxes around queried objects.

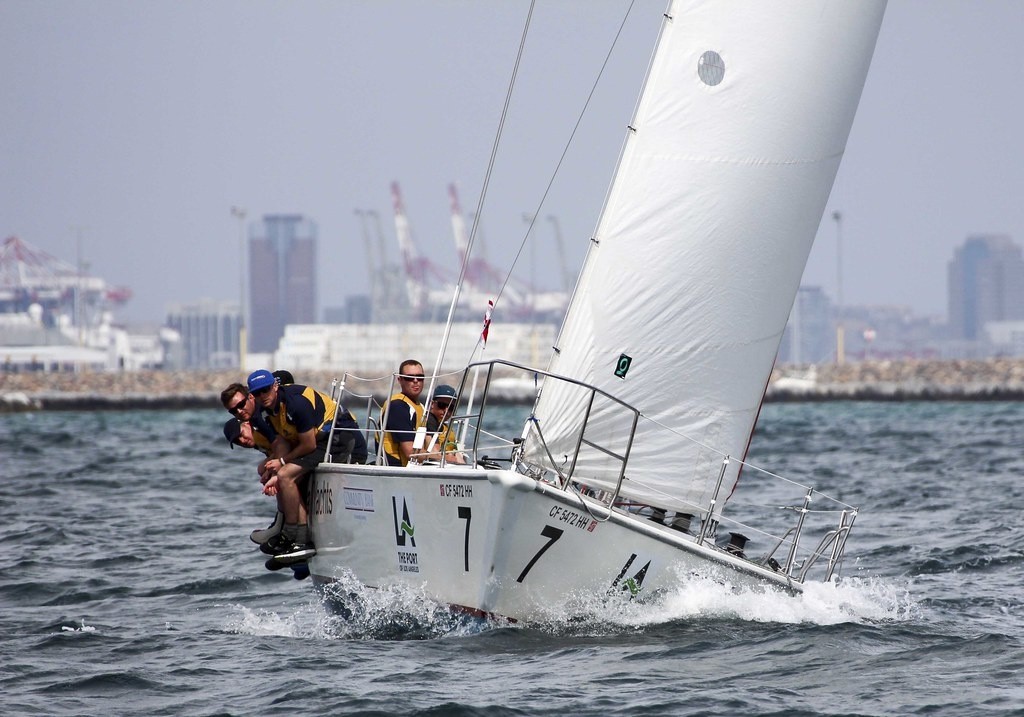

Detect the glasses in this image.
[252,386,270,397]
[402,374,425,382]
[433,400,454,411]
[228,396,248,415]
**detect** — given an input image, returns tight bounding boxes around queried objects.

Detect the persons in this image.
[375,359,440,467]
[426,384,466,465]
[221,369,368,581]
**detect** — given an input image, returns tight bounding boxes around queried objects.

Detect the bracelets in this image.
[280,458,285,465]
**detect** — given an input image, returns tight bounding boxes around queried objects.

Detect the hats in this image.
[272,371,294,386]
[248,370,274,392]
[224,418,244,449]
[433,385,458,400]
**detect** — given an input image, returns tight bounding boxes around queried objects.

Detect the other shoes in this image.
[265,558,292,570]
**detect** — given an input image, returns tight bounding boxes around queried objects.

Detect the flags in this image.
[477,301,493,349]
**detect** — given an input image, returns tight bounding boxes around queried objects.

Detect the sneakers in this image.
[273,543,317,562]
[260,533,292,555]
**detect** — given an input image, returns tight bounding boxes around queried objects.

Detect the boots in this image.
[250,510,285,544]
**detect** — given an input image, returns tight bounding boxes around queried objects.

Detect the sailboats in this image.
[306,0,888,635]
